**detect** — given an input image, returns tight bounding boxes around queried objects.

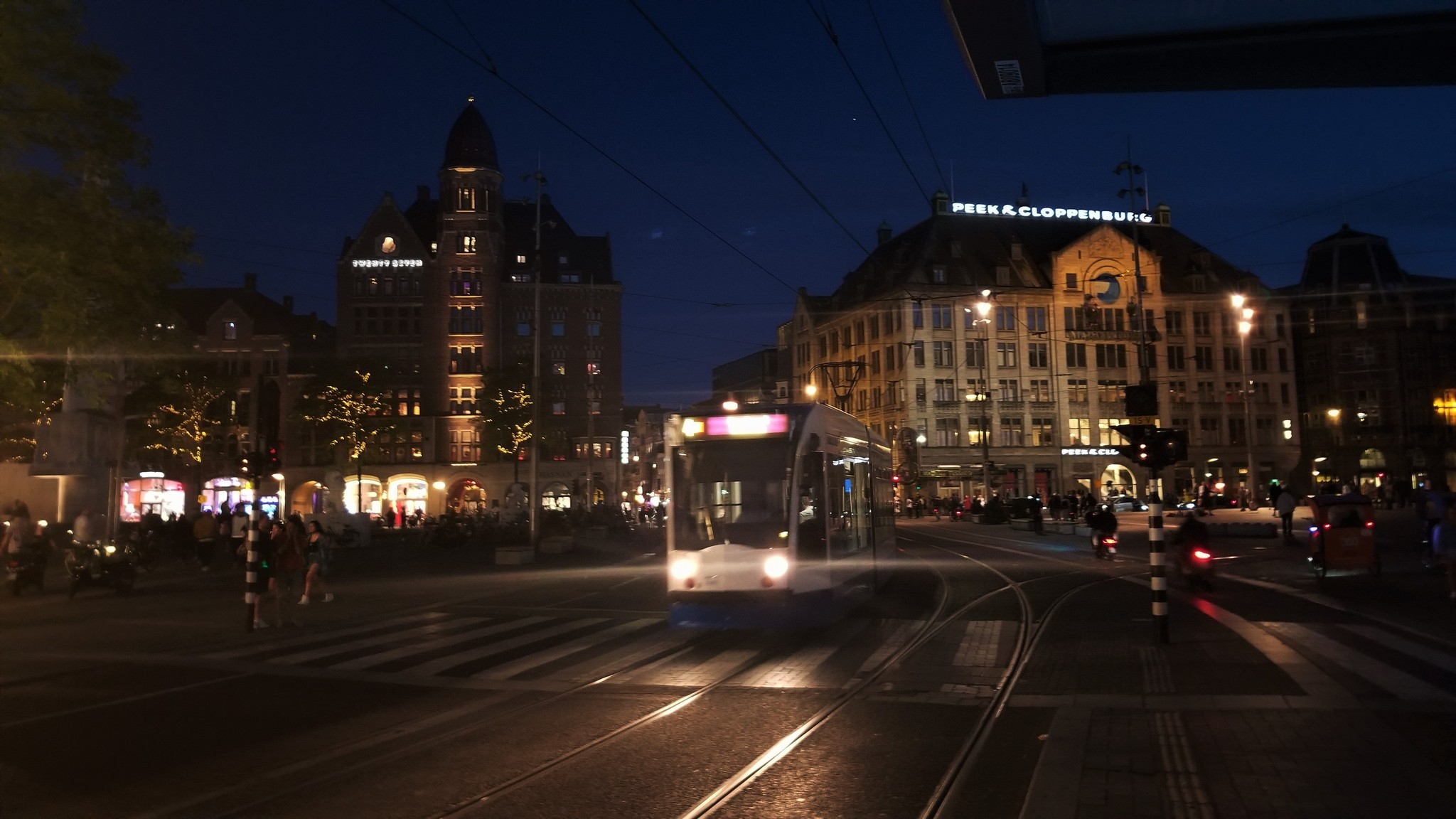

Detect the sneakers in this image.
[253,618,269,630]
[297,595,310,605]
[322,592,334,602]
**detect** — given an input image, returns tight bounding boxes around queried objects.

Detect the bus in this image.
[664,401,892,639]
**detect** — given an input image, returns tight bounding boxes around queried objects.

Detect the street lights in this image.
[970,288,993,509]
[1230,293,1260,511]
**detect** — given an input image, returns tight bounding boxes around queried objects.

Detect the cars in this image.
[1095,496,1146,512]
[1177,493,1238,510]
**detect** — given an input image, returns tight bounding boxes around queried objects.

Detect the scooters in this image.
[1089,525,1119,561]
[1,517,157,601]
[930,504,963,523]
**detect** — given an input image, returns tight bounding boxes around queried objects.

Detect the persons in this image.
[739,464,1409,551]
[245,510,272,630]
[1419,493,1446,575]
[0,491,262,581]
[286,514,310,593]
[384,490,667,543]
[298,521,335,605]
[268,520,289,604]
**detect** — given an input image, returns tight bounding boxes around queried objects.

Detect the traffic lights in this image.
[236,436,285,481]
